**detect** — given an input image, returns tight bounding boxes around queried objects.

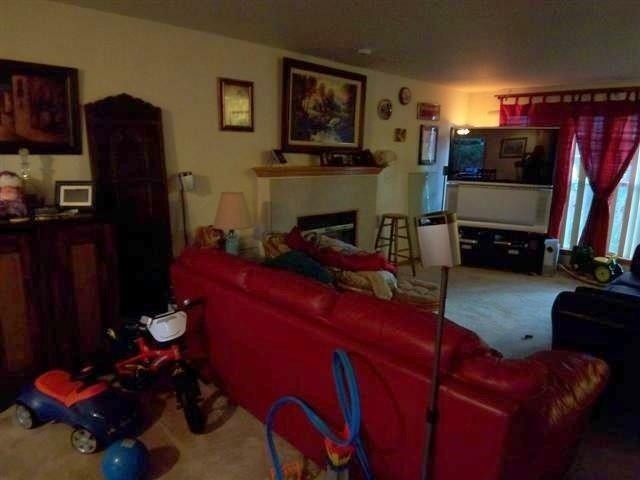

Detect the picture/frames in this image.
[418,125,438,164]
[500,137,528,158]
[218,77,254,130]
[281,56,367,155]
[0,59,83,155]
[55,181,96,210]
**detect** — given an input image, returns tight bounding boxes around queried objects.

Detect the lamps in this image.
[214,191,253,254]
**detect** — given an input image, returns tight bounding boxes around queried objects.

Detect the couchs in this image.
[552,244,639,362]
[167,244,610,479]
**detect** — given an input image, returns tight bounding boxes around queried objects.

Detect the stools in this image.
[374,213,416,275]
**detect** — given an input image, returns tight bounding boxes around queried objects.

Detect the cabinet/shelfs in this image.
[84,93,170,317]
[0,222,121,410]
